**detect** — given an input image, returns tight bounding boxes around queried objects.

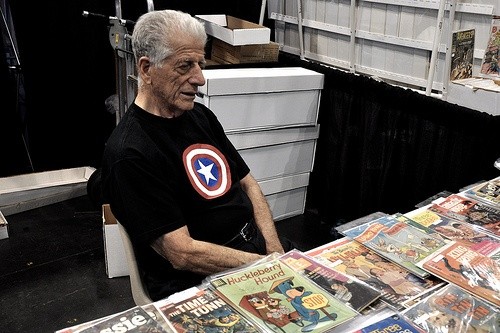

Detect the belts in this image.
[223,218,257,248]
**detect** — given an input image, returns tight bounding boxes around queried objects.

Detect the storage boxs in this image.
[195,14,272,46]
[102,204,132,279]
[208,37,279,66]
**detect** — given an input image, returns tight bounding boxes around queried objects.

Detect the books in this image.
[55,176,500,333]
[450,19,500,80]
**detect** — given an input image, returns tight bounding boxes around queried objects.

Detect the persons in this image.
[341,261,373,280]
[360,251,404,272]
[369,268,425,293]
[99,10,286,301]
[452,224,487,241]
